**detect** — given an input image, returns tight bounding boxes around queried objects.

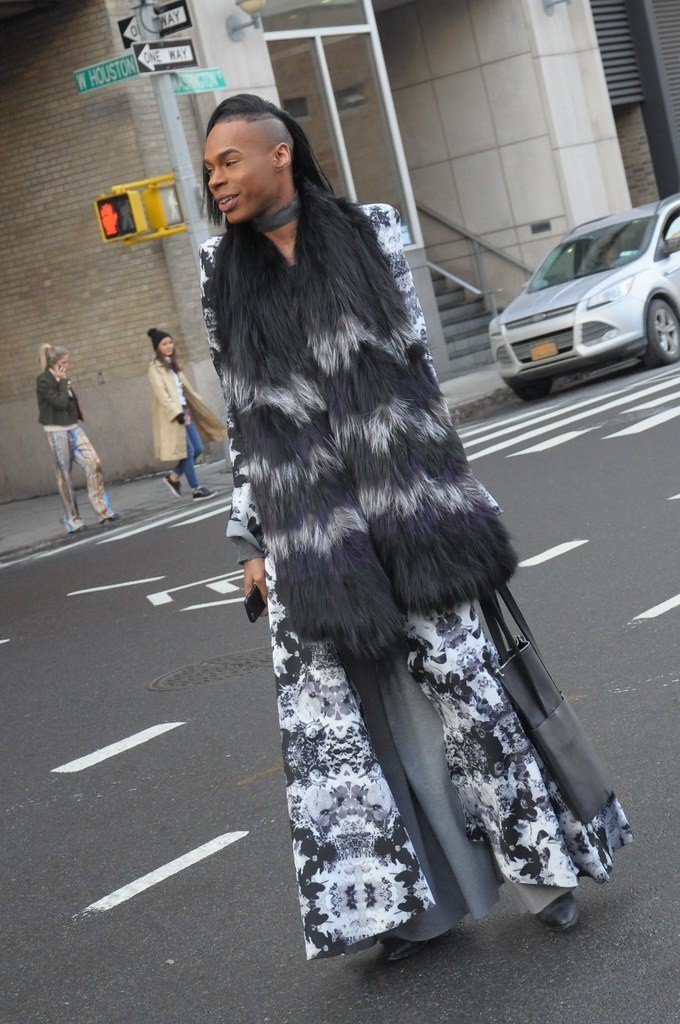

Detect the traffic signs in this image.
[116,1,195,49]
[130,36,201,76]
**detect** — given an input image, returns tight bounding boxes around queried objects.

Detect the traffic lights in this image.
[94,189,148,243]
[142,180,184,232]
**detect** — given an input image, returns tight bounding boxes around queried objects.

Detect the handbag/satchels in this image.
[477,583,613,825]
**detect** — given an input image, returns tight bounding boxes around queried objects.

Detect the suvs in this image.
[489,193,680,402]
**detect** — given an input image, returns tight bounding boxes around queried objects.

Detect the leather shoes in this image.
[381,936,427,960]
[535,891,578,931]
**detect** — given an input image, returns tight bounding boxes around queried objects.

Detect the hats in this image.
[147,328,171,351]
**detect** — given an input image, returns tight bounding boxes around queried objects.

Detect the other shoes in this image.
[69,525,86,533]
[100,513,119,524]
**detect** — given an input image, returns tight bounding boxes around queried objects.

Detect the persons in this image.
[199,92,640,966]
[146,328,228,502]
[35,342,119,533]
[599,237,622,269]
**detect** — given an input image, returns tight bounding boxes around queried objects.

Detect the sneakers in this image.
[163,472,181,498]
[193,487,218,502]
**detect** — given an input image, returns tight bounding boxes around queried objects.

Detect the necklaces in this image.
[252,195,300,234]
